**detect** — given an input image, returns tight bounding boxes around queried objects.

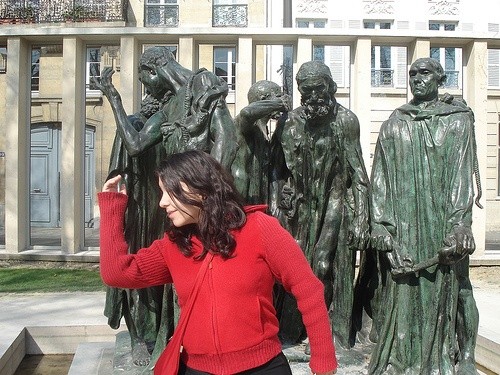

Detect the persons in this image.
[92,46,483,375]
[98,149,338,375]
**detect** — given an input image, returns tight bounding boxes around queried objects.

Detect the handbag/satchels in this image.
[153,336,183,375]
